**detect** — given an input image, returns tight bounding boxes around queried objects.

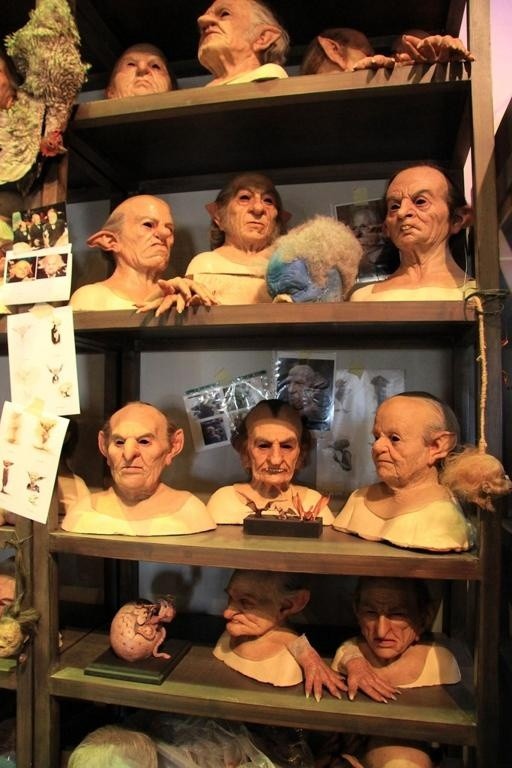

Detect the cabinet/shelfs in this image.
[0,0,505,768]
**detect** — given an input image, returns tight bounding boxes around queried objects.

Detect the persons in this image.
[348,164,480,302]
[59,401,217,534]
[196,0,291,86]
[5,207,70,283]
[301,23,475,74]
[205,398,337,525]
[341,734,434,768]
[331,575,461,704]
[331,391,477,552]
[151,172,295,304]
[65,195,217,311]
[212,567,349,703]
[102,40,180,100]
[68,726,158,767]
